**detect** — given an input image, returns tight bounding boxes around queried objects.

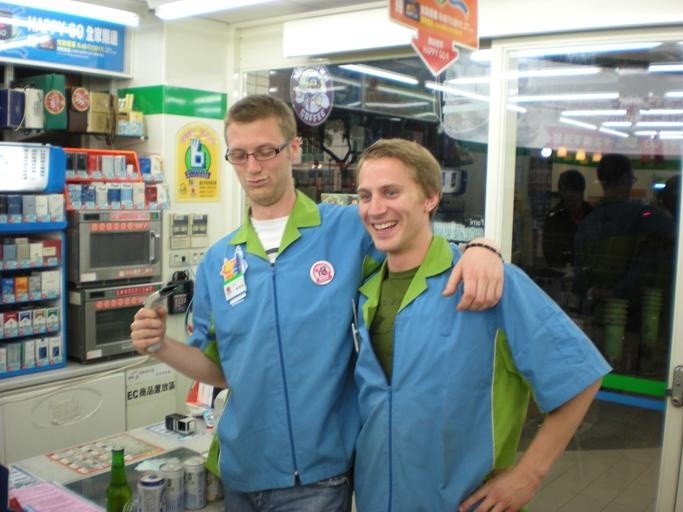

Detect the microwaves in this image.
[66,281,166,364]
[68,207,165,285]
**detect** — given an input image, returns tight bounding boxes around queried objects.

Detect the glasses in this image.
[626,175,638,186]
[225,142,288,164]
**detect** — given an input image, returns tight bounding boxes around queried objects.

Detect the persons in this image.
[353,138,614,512]
[542,153,681,374]
[130,94,504,512]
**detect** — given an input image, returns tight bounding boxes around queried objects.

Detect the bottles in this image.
[105,445,134,512]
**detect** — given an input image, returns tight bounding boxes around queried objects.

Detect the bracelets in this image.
[464,243,502,258]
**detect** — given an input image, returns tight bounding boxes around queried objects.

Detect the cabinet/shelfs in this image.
[62,146,149,214]
[0,194,66,383]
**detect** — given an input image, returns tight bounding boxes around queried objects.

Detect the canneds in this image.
[136,453,222,512]
